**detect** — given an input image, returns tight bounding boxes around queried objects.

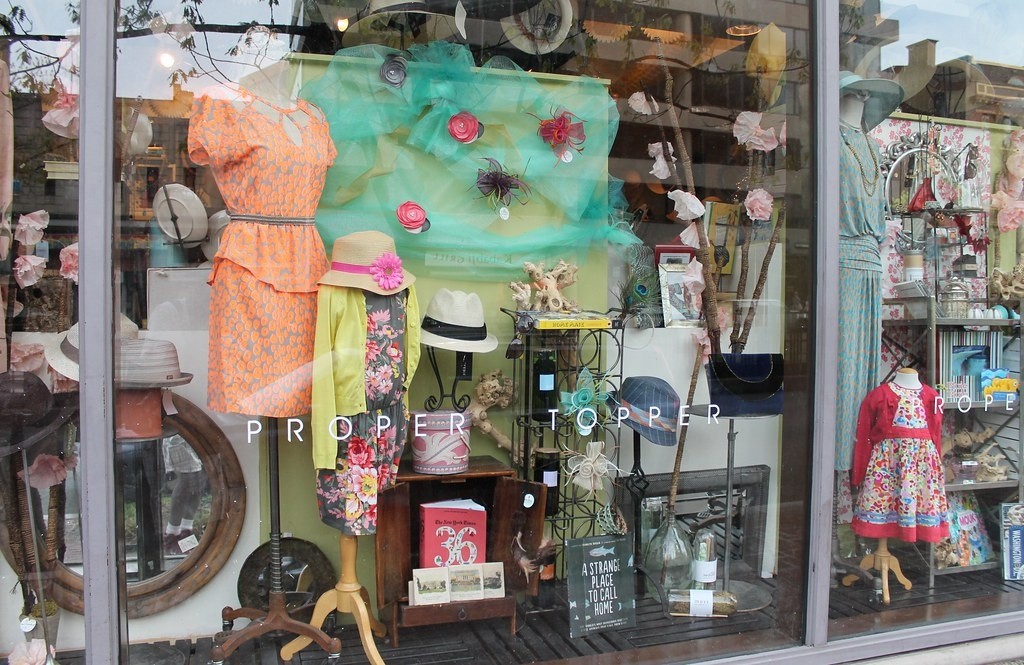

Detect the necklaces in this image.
[841,121,879,197]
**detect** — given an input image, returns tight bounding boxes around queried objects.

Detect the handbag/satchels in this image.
[705,351,785,418]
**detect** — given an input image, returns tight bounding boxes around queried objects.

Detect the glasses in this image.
[950,142,979,181]
[500,307,534,359]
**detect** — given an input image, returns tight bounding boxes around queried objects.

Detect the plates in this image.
[202,209,234,263]
[153,183,208,248]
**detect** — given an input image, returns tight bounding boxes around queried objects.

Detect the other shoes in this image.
[164,529,193,551]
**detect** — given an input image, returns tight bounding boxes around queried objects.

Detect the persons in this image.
[851,368,950,541]
[187,40,337,418]
[834,71,904,471]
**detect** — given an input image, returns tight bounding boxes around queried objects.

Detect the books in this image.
[420,498,487,568]
[408,563,505,606]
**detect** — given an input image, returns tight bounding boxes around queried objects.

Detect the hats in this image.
[340,0,464,52]
[603,376,680,446]
[500,0,573,55]
[420,287,498,353]
[316,231,416,295]
[838,70,905,134]
[43,312,138,383]
[904,47,996,116]
[114,338,194,387]
[853,33,936,104]
